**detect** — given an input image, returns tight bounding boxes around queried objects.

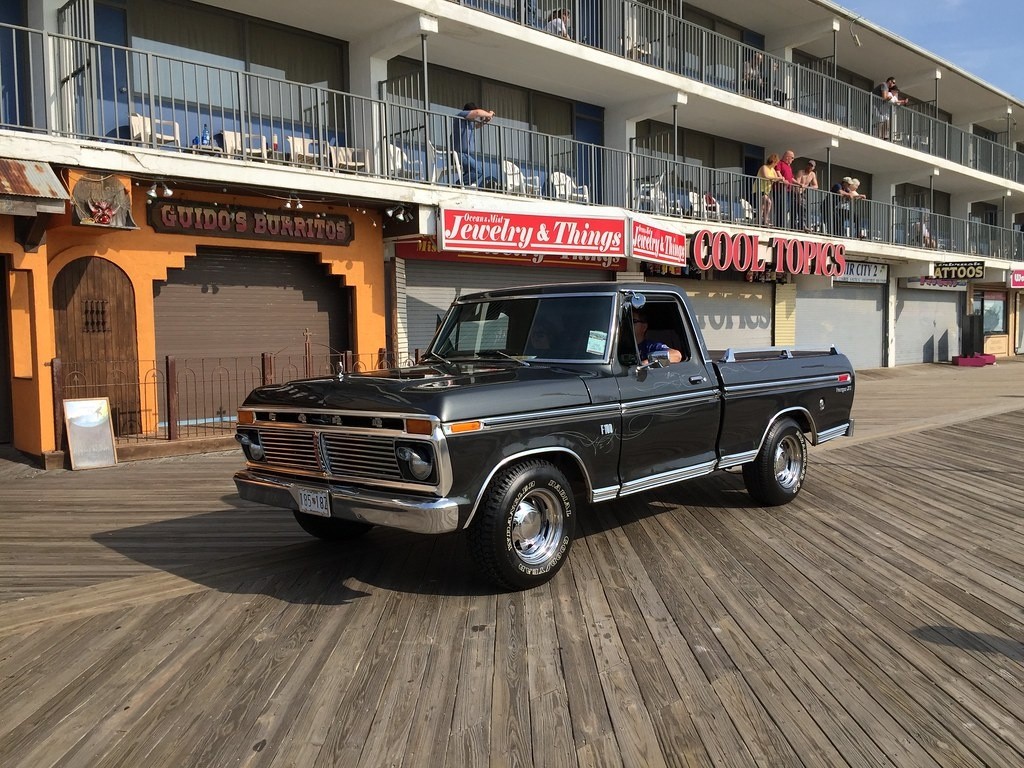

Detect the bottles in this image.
[273,134,278,150]
[202,124,210,145]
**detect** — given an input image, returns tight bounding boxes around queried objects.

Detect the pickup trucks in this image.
[231,280,856,588]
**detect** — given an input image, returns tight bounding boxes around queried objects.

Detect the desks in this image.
[191,145,223,158]
[676,207,691,219]
[257,148,283,166]
[485,180,507,195]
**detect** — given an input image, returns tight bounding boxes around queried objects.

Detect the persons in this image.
[742,52,764,99]
[512,0,571,40]
[868,77,908,141]
[447,103,495,188]
[914,215,935,248]
[751,150,867,239]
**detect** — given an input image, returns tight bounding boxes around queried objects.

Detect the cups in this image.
[192,136,203,144]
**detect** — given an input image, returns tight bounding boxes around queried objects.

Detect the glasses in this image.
[633,318,642,324]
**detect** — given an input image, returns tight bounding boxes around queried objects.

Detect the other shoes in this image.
[804,225,811,232]
[762,222,773,227]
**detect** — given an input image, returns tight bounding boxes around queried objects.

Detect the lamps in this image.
[147,180,174,198]
[849,19,860,48]
[388,205,405,221]
[286,192,304,208]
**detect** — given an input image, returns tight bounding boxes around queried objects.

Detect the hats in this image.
[808,160,816,166]
[843,177,854,185]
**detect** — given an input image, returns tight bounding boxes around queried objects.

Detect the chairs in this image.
[503,160,542,199]
[389,144,424,184]
[222,131,267,164]
[428,139,477,191]
[911,223,926,248]
[552,172,589,205]
[130,112,181,155]
[634,172,682,218]
[626,42,928,150]
[526,321,562,360]
[287,136,370,172]
[689,192,769,226]
